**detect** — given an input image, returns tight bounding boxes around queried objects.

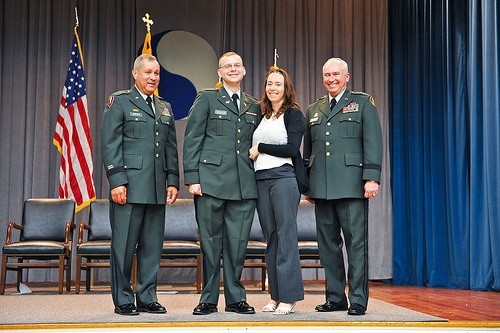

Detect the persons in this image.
[304,57,384,315]
[183,51,267,315]
[248,65,309,316]
[98,54,181,316]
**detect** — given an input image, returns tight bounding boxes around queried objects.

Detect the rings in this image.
[372,193,376,198]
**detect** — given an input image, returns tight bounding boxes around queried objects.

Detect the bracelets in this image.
[369,180,376,183]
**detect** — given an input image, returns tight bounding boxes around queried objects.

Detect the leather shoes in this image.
[192,302,218,314]
[114,303,139,315]
[224,301,255,314]
[347,303,365,315]
[318,301,348,311]
[136,302,167,313]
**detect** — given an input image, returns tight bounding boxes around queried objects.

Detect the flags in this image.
[133,30,160,96]
[52,22,98,217]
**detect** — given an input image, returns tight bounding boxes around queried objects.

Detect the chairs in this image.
[0,197,77,294]
[219,208,268,291]
[75,198,139,295]
[296,199,326,285]
[156,198,203,294]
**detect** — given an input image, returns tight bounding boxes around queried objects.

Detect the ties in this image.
[330,98,337,111]
[232,94,240,113]
[145,97,154,115]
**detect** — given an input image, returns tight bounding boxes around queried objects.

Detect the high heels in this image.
[261,298,280,311]
[273,299,297,314]
